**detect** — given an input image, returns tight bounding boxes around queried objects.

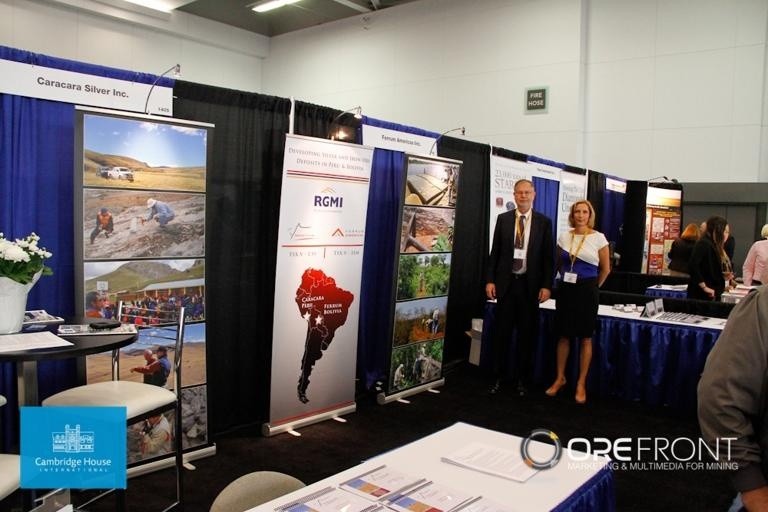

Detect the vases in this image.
[1,264,44,336]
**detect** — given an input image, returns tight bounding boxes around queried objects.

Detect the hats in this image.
[147,199,156,207]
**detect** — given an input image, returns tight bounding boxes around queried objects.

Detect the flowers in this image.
[0,229,56,288]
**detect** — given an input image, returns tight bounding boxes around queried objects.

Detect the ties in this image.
[513,216,525,270]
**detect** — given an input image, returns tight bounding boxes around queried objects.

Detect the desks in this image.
[482,299,729,410]
[0,314,140,456]
[244,419,617,511]
[644,284,688,299]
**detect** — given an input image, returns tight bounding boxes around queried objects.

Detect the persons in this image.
[143,199,176,227]
[86,292,204,325]
[144,350,158,384]
[137,414,171,459]
[697,285,766,512]
[484,180,557,401]
[545,201,610,405]
[90,208,113,244]
[742,224,767,295]
[129,346,171,389]
[393,307,438,391]
[665,216,735,300]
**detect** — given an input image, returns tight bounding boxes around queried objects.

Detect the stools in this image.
[0,452,38,512]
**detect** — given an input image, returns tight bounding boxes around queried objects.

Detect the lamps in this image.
[429,126,466,154]
[645,174,669,187]
[142,63,183,115]
[324,104,364,140]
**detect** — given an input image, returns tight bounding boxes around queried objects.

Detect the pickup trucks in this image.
[107,166,135,182]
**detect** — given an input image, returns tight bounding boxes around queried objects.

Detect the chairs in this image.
[40,299,187,511]
[209,468,308,511]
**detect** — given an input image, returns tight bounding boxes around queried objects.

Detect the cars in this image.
[95,166,111,178]
